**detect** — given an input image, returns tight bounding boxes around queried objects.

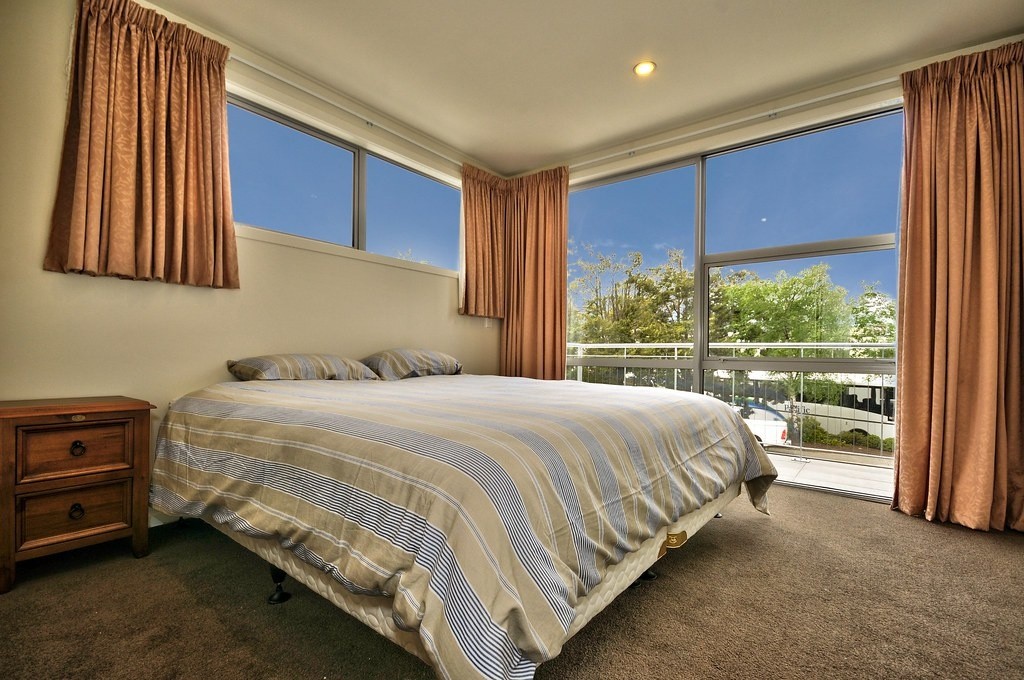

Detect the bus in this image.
[714,370,896,440]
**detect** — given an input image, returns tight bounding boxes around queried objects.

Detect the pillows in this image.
[227,353,382,381]
[357,347,463,380]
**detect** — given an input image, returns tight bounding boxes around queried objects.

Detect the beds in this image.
[147,369,778,680]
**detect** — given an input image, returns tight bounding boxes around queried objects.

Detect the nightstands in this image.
[0,396,158,595]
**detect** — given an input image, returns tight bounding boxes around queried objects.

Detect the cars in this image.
[725,396,793,448]
